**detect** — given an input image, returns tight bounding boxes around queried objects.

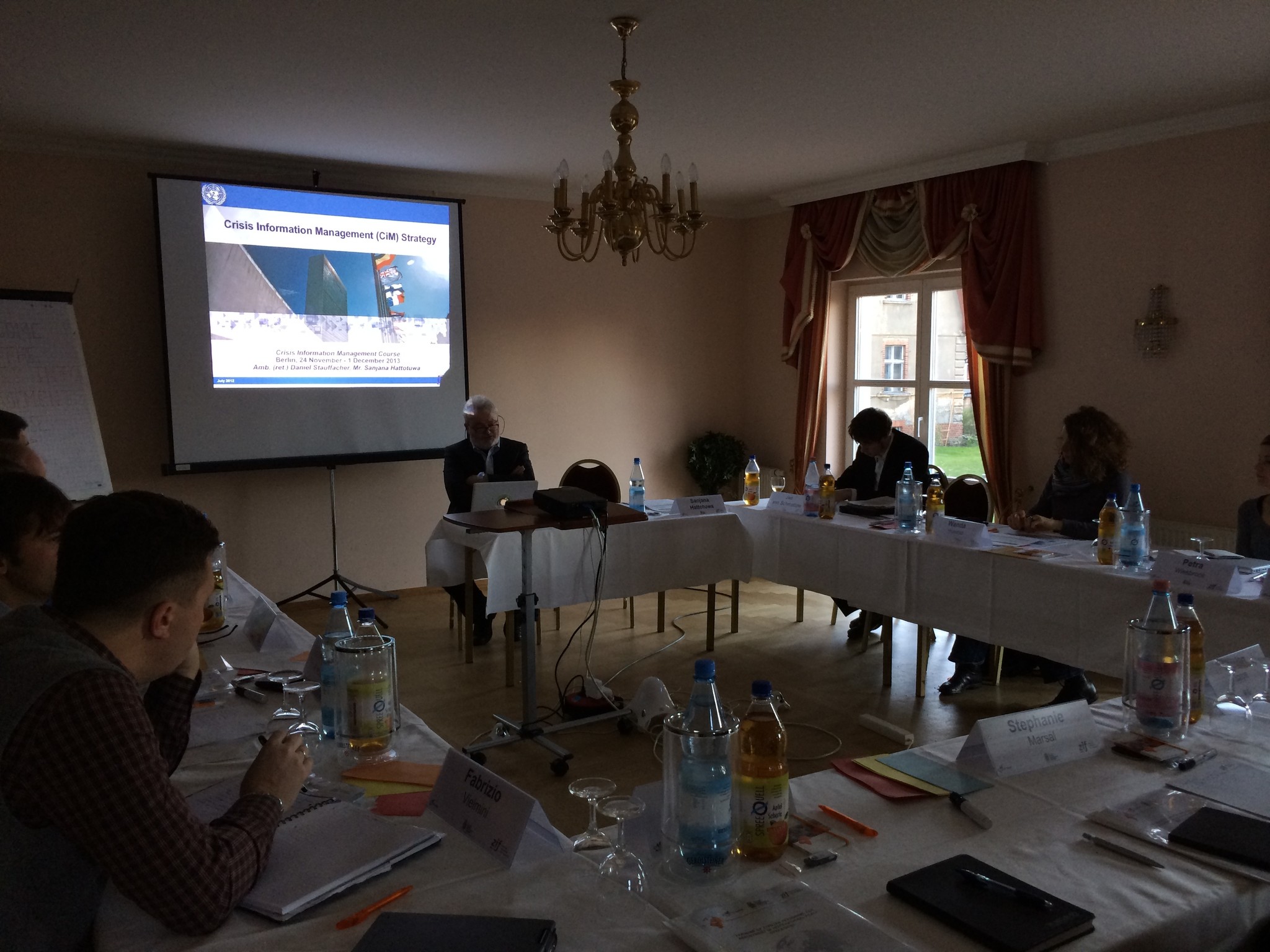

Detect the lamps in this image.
[537,17,709,269]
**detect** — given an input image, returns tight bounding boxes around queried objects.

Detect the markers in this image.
[1179,748,1218,770]
[235,684,268,704]
[949,791,993,830]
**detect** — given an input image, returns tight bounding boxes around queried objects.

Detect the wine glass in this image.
[1248,656,1270,735]
[743,478,744,501]
[282,680,326,765]
[1091,518,1101,556]
[568,779,618,863]
[266,671,306,736]
[1189,535,1213,560]
[593,796,651,899]
[1210,655,1260,738]
[771,476,786,492]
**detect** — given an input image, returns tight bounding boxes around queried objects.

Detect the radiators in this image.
[737,467,787,505]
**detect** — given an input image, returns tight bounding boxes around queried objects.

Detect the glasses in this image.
[467,417,499,434]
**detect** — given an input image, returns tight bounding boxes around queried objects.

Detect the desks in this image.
[52,487,1269,952]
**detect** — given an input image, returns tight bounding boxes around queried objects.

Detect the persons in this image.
[0,474,75,617]
[1,489,313,952]
[833,406,931,638]
[939,404,1132,706]
[1234,434,1270,563]
[0,408,46,480]
[424,394,538,644]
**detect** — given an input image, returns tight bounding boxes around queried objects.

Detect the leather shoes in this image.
[473,596,498,646]
[938,665,983,695]
[503,609,522,641]
[1039,677,1100,707]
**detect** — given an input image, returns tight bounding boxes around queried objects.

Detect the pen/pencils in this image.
[1249,571,1268,582]
[1082,832,1166,869]
[818,804,878,838]
[232,672,267,685]
[987,528,998,533]
[258,735,308,792]
[961,868,1054,909]
[336,885,414,931]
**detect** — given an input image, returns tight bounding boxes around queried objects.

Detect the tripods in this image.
[274,467,400,628]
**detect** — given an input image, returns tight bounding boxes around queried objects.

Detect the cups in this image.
[895,479,923,534]
[665,710,744,883]
[1125,614,1191,743]
[1118,504,1154,567]
[334,634,403,768]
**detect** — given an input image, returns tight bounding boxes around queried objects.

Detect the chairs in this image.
[551,458,636,630]
[923,458,995,644]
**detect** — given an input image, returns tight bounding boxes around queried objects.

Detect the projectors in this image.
[532,485,608,520]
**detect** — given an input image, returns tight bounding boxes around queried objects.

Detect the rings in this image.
[297,748,306,761]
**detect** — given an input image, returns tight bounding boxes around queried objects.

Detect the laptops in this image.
[471,481,539,513]
[1227,557,1270,574]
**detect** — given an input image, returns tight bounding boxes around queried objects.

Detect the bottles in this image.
[629,457,646,514]
[1119,483,1148,567]
[679,660,733,870]
[744,456,760,505]
[345,604,399,749]
[803,457,820,518]
[1136,576,1181,734]
[926,473,944,531]
[737,681,797,858]
[320,592,357,739]
[201,544,225,633]
[899,462,916,528]
[819,463,835,519]
[1174,591,1206,727]
[1098,492,1121,569]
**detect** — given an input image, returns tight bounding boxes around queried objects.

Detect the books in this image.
[178,770,441,925]
[885,854,1096,952]
[1171,548,1245,561]
[1168,806,1269,874]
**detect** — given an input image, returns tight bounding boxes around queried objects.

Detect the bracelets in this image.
[242,791,285,820]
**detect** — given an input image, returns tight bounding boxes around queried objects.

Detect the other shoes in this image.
[847,609,882,639]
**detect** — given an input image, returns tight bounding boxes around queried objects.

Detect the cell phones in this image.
[1169,806,1270,873]
